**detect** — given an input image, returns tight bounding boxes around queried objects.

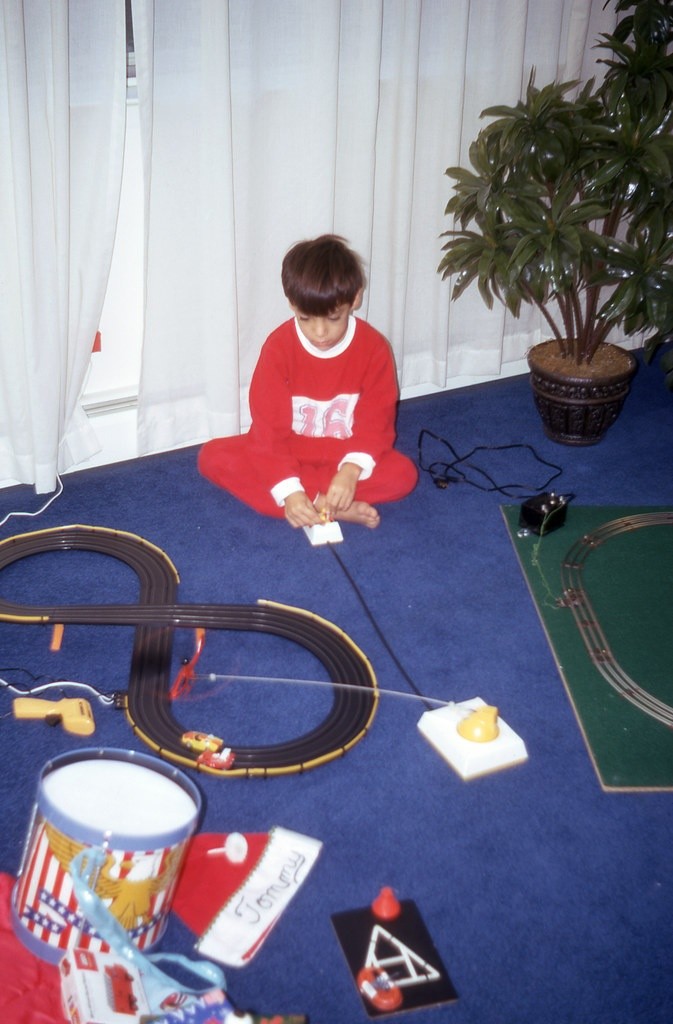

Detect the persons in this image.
[198,233,417,529]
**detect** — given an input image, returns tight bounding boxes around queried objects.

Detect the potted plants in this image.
[436,0,673,445]
